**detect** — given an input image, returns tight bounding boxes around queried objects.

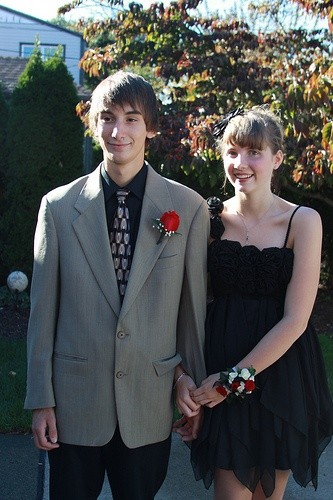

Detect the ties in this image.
[109,189,133,298]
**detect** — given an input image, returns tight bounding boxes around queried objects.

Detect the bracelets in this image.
[174,371,186,386]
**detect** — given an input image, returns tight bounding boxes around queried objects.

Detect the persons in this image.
[174,107,332,500]
[23,70,211,500]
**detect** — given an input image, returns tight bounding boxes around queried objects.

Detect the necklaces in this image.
[232,196,275,241]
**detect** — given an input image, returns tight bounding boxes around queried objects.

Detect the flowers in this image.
[152,211,183,246]
[211,367,259,400]
